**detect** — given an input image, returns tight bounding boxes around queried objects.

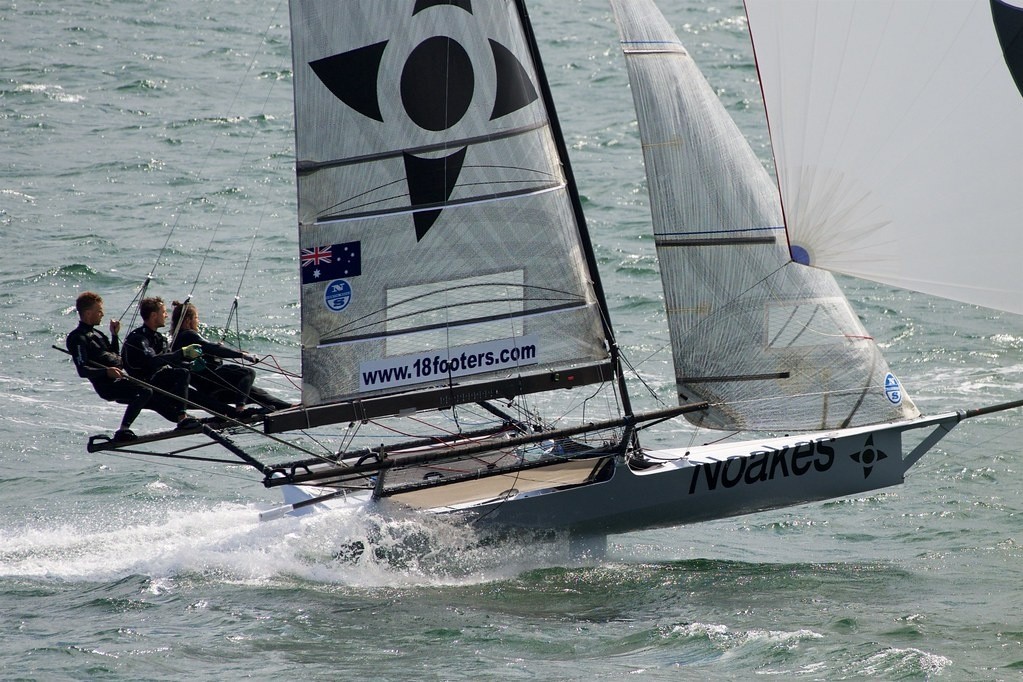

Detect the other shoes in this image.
[232,409,259,420]
[177,418,201,430]
[291,404,303,407]
[114,430,138,442]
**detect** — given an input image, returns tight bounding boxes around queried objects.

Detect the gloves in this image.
[244,353,259,364]
[182,344,202,358]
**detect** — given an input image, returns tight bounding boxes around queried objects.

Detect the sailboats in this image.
[51,1,1023,560]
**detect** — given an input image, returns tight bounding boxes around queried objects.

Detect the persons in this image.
[120,295,276,428]
[170,299,301,415]
[64,291,207,440]
